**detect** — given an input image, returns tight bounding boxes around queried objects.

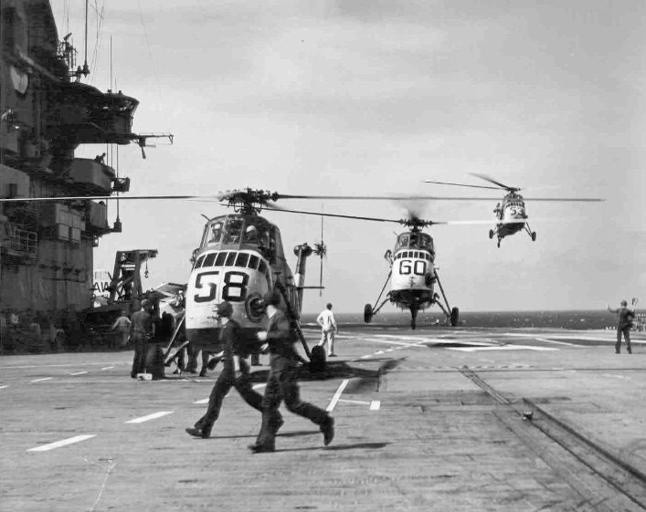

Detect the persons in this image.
[112,310,132,347]
[185,301,284,439]
[606,300,635,354]
[206,224,225,242]
[316,303,338,357]
[244,224,266,250]
[246,291,334,452]
[0,306,89,349]
[127,299,156,378]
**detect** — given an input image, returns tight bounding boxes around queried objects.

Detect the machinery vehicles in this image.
[80,241,162,348]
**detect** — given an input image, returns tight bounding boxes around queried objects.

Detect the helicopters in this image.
[218,202,571,331]
[0,186,607,379]
[420,168,606,248]
